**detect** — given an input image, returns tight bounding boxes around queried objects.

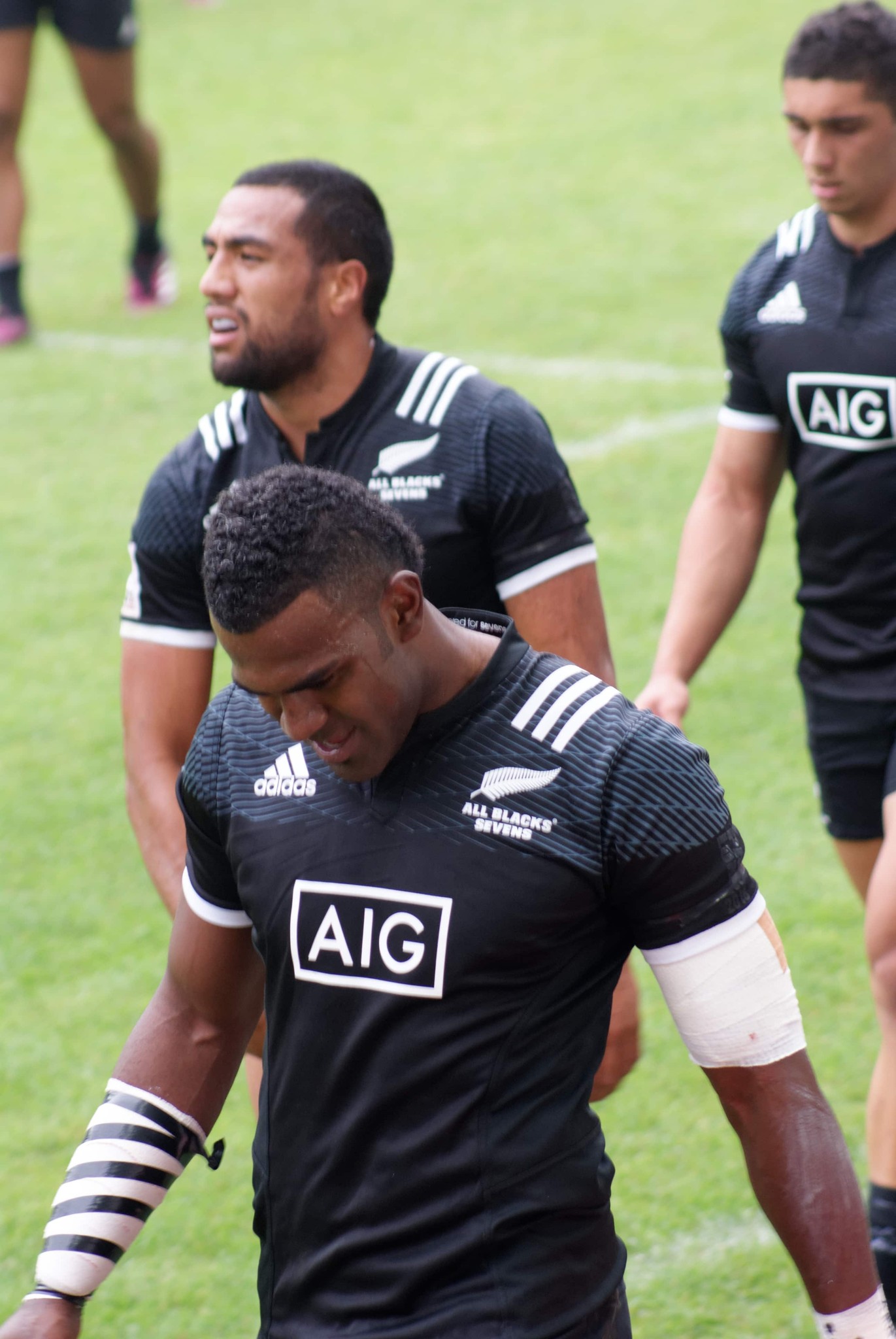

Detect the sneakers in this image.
[0,261,24,341]
[129,245,168,303]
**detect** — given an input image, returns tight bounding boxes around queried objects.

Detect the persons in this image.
[0,0,176,348]
[617,0,896,1318]
[0,467,896,1339]
[121,163,644,1105]
